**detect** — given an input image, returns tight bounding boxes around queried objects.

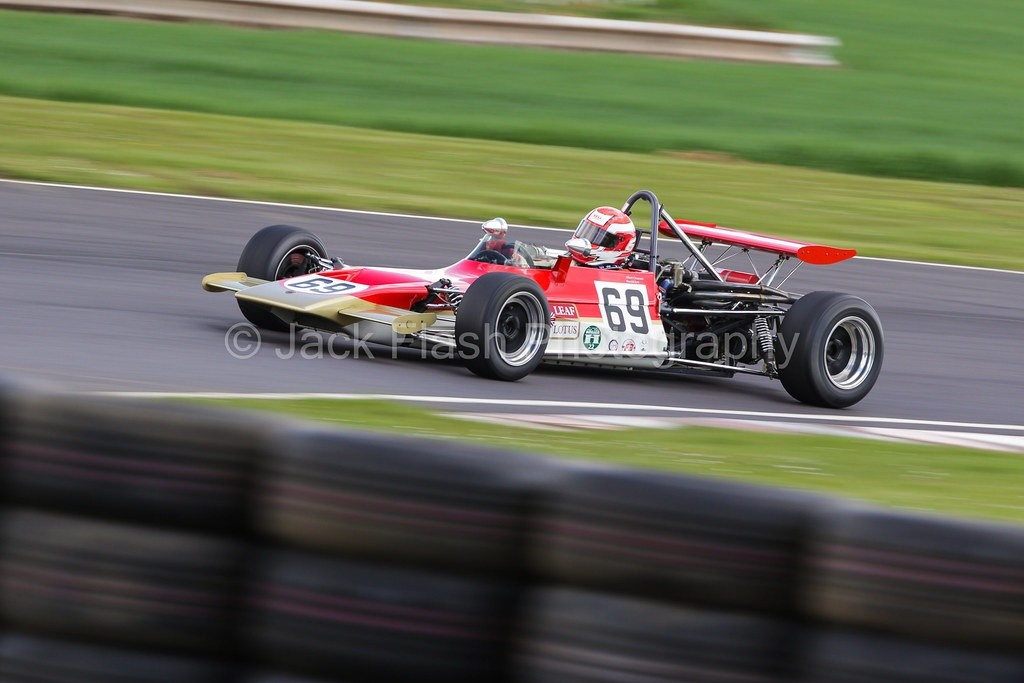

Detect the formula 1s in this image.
[200,189,887,409]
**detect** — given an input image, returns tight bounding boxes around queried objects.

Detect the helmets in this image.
[569,206,636,266]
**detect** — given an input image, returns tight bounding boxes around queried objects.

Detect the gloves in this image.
[485,233,515,258]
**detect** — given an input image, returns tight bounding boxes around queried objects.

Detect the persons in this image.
[481,206,637,268]
